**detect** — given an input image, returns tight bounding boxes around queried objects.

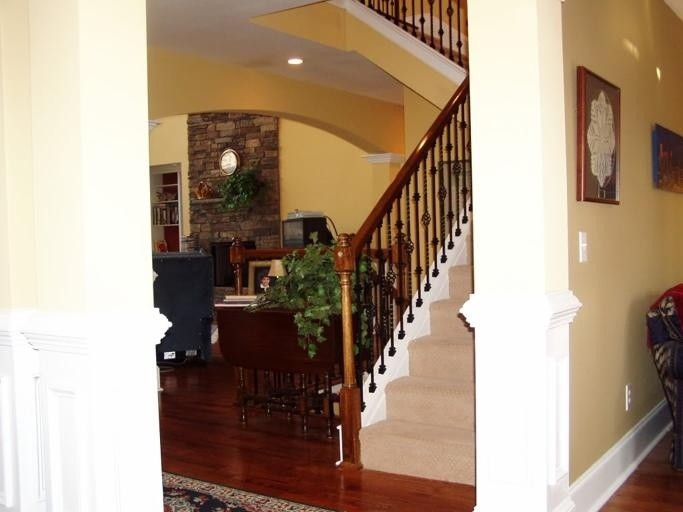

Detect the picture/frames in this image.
[576,66,622,206]
[248,261,284,299]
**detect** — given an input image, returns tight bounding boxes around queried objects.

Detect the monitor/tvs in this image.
[282,217,335,248]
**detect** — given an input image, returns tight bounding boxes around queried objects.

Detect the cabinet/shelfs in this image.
[150,163,185,253]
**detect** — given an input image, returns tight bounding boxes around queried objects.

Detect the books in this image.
[151,205,179,225]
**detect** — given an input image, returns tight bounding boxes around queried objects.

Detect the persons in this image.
[256,271,272,294]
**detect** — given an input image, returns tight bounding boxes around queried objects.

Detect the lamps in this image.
[268,259,289,279]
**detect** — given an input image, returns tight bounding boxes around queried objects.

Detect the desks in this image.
[212,299,371,439]
